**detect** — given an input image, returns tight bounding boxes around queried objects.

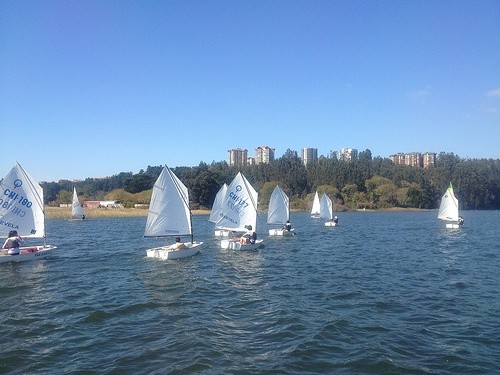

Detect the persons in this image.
[282,220,291,230]
[170,237,187,251]
[334,216,338,224]
[458,216,464,225]
[2,230,24,255]
[241,225,257,244]
[82,215,85,220]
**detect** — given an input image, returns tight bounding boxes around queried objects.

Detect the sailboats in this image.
[142,164,204,262]
[437,181,465,230]
[266,183,295,238]
[208,172,265,251]
[0,161,57,263]
[65,186,93,221]
[310,188,341,228]
